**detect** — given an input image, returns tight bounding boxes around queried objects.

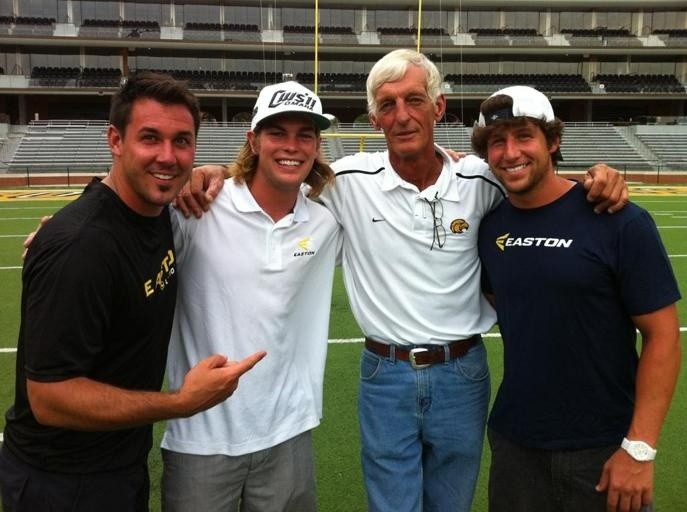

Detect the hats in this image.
[477,85,564,162]
[250,79,332,135]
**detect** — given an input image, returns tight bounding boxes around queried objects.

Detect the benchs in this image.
[8,123,687,176]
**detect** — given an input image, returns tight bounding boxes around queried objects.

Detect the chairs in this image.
[0,15,687,100]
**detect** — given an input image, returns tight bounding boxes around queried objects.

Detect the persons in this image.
[445,85,682,512]
[1,68,267,511]
[19,81,341,512]
[162,49,630,511]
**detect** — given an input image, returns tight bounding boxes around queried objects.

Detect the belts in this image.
[364,335,482,371]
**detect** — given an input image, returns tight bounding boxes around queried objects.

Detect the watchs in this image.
[619,436,655,464]
